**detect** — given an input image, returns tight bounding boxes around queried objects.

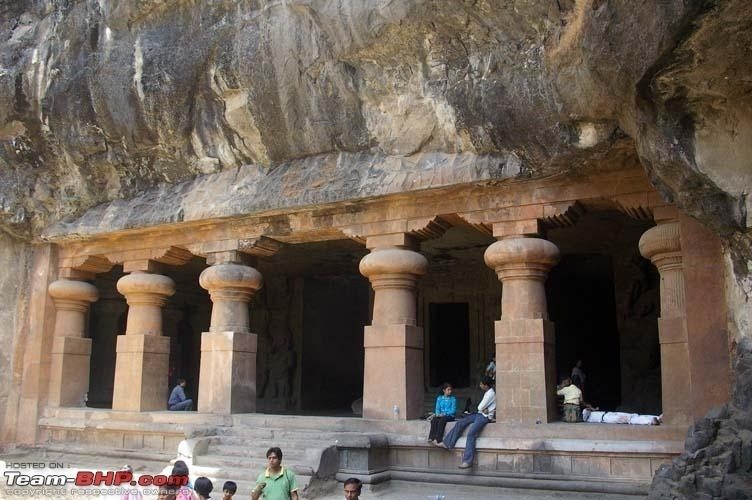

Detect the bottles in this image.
[392,404,399,421]
[427,494,445,500]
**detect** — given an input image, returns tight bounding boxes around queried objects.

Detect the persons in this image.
[427,352,496,469]
[168,378,193,411]
[120,447,299,500]
[344,478,362,500]
[556,359,584,423]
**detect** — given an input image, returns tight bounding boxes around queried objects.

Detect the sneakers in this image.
[427,439,448,449]
[458,463,473,469]
